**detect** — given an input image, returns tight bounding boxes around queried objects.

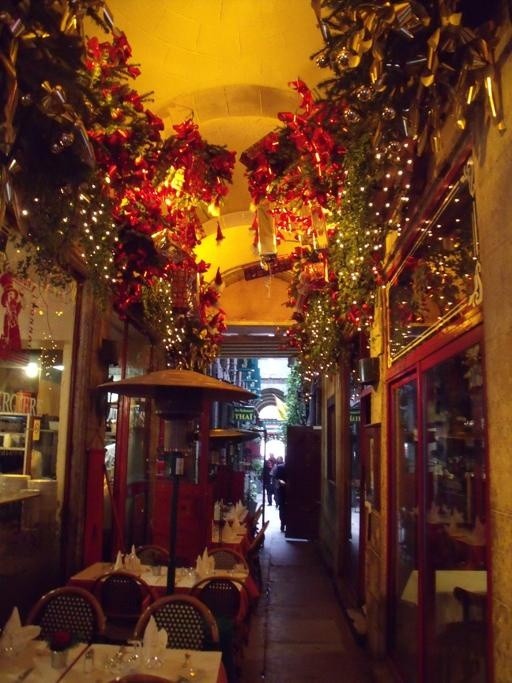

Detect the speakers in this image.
[257,201,277,259]
[310,208,329,251]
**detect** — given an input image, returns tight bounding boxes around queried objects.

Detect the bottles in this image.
[178,653,196,681]
[82,653,93,673]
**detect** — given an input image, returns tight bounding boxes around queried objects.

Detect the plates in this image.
[1,474,59,510]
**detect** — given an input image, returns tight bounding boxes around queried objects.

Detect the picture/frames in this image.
[361,422,381,512]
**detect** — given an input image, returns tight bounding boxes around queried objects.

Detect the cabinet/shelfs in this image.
[1,411,42,475]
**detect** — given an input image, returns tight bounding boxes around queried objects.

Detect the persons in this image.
[273,479,286,532]
[270,456,286,506]
[267,453,277,472]
[263,459,275,506]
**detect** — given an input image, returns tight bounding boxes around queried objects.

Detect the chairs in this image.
[246,503,269,584]
[25,544,252,650]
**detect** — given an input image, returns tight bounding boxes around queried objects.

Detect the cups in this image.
[51,651,67,669]
